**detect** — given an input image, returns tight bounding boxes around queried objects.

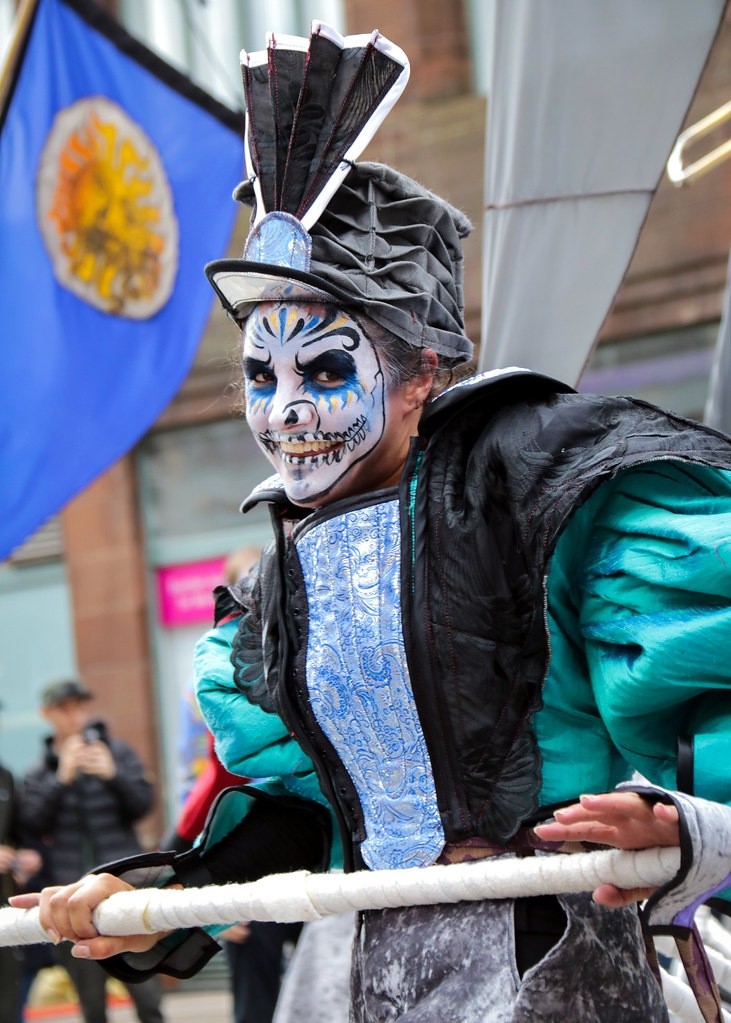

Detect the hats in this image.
[203,18,477,364]
[44,683,95,706]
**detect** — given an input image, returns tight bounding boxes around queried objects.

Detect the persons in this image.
[8,159,731,1023]
[1,680,304,1023]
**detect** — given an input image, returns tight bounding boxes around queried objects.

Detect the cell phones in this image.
[83,728,101,745]
